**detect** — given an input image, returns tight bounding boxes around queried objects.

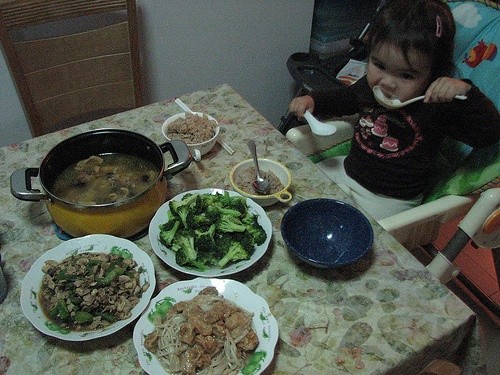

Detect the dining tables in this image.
[0,81,475,375]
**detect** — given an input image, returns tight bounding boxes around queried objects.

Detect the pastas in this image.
[154,296,249,375]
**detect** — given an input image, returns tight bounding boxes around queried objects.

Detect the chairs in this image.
[287,0,500,285]
[0,0,143,137]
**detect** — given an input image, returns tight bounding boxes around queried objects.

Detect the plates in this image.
[20,234,157,342]
[133,277,279,375]
[148,188,272,279]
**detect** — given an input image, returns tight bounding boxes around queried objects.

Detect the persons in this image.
[289,0,500,221]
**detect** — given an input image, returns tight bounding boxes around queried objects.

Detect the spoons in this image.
[373,86,467,108]
[304,111,336,137]
[246,140,272,196]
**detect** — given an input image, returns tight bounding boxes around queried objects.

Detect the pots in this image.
[8,126,191,239]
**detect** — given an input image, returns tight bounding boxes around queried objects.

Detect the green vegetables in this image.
[50,259,147,323]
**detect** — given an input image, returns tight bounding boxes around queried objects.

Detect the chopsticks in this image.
[173,97,235,156]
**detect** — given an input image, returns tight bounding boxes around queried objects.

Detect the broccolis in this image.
[158,190,267,272]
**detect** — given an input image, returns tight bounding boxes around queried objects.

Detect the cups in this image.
[0,266,9,304]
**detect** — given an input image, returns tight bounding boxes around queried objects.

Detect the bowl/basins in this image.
[162,112,220,161]
[229,159,294,206]
[280,198,374,270]
